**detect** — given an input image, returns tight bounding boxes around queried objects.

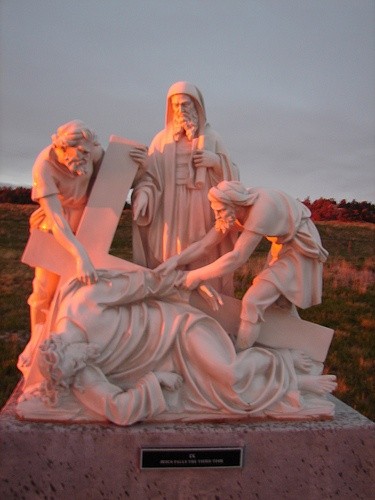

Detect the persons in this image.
[147,177,330,356]
[133,81,242,300]
[38,265,340,427]
[20,119,150,339]
